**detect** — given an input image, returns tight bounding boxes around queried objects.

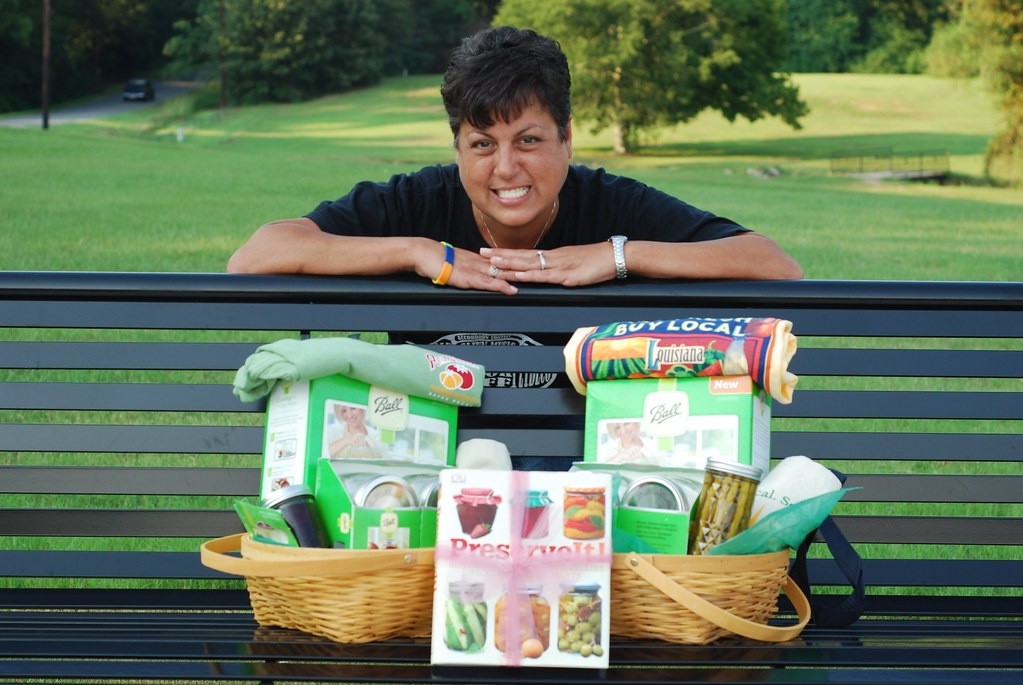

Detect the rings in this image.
[537,251,546,270]
[490,266,499,277]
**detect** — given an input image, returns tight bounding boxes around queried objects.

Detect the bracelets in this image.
[431,240,455,285]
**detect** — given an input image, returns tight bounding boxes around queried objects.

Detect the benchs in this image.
[1,266,1023,685]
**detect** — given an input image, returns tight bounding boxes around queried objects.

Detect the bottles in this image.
[562,488,605,540]
[557,583,603,654]
[400,472,439,507]
[495,582,550,652]
[261,483,332,549]
[621,471,703,510]
[445,581,489,651]
[336,473,418,508]
[521,490,550,538]
[692,456,763,555]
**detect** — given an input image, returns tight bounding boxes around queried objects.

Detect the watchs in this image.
[608,235,628,278]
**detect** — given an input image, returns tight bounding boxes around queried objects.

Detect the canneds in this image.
[522,489,552,539]
[496,584,551,655]
[556,581,604,651]
[443,581,489,651]
[688,456,762,557]
[562,486,609,541]
[262,484,333,549]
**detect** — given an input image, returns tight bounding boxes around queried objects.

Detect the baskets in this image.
[200,534,434,642]
[614,552,812,645]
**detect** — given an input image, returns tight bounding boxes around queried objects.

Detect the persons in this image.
[229,27,804,473]
[602,423,661,467]
[327,402,382,458]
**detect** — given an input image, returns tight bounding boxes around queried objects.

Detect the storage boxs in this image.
[258,373,458,508]
[585,376,772,481]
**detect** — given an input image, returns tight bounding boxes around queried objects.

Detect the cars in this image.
[121,77,153,101]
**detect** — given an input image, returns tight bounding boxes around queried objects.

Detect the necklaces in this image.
[477,199,556,249]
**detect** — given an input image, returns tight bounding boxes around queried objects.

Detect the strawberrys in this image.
[470,523,492,538]
